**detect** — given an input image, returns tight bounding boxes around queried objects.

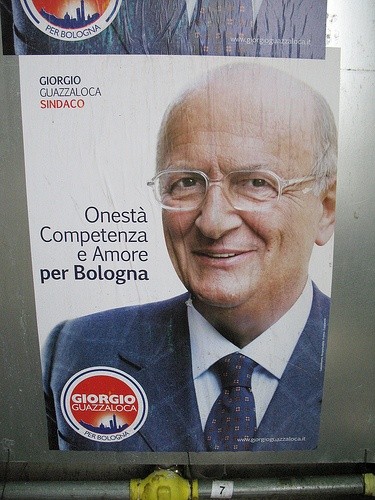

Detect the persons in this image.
[38,58,337,450]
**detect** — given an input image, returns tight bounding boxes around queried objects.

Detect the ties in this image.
[203,353,259,451]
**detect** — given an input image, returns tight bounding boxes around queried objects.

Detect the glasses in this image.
[147,168,327,213]
